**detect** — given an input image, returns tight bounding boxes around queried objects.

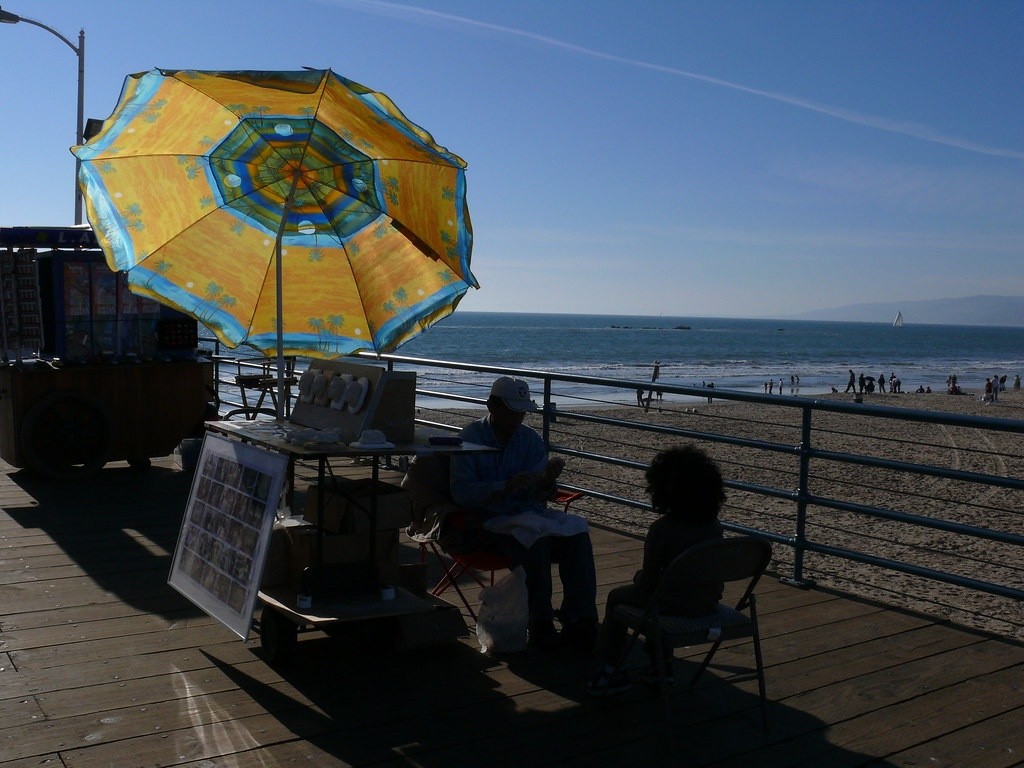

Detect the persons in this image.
[636,388,644,407]
[946,374,952,388]
[769,379,773,394]
[916,385,924,393]
[985,373,1021,405]
[844,370,856,392]
[859,373,866,394]
[831,387,838,393]
[926,386,931,393]
[692,384,695,387]
[297,372,362,407]
[791,376,794,382]
[952,374,957,386]
[779,378,783,395]
[764,381,767,394]
[703,381,705,387]
[795,375,800,384]
[448,377,601,665]
[864,381,875,394]
[889,372,901,393]
[707,383,714,404]
[878,374,886,393]
[585,445,728,693]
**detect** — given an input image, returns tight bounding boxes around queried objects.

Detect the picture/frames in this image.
[166,431,288,642]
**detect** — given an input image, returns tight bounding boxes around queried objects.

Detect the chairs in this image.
[406,457,585,623]
[237,355,298,420]
[599,538,772,762]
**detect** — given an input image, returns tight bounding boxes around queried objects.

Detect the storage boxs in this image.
[264,515,401,604]
[303,479,417,533]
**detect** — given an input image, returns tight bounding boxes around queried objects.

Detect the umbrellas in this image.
[865,376,875,382]
[68,65,480,422]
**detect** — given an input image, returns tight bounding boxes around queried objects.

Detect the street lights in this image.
[0,3,87,226]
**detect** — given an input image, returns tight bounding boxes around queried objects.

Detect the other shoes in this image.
[587,670,631,696]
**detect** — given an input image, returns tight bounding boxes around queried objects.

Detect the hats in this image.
[490,377,539,412]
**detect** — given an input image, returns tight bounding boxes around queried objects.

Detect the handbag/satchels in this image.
[476,565,529,655]
